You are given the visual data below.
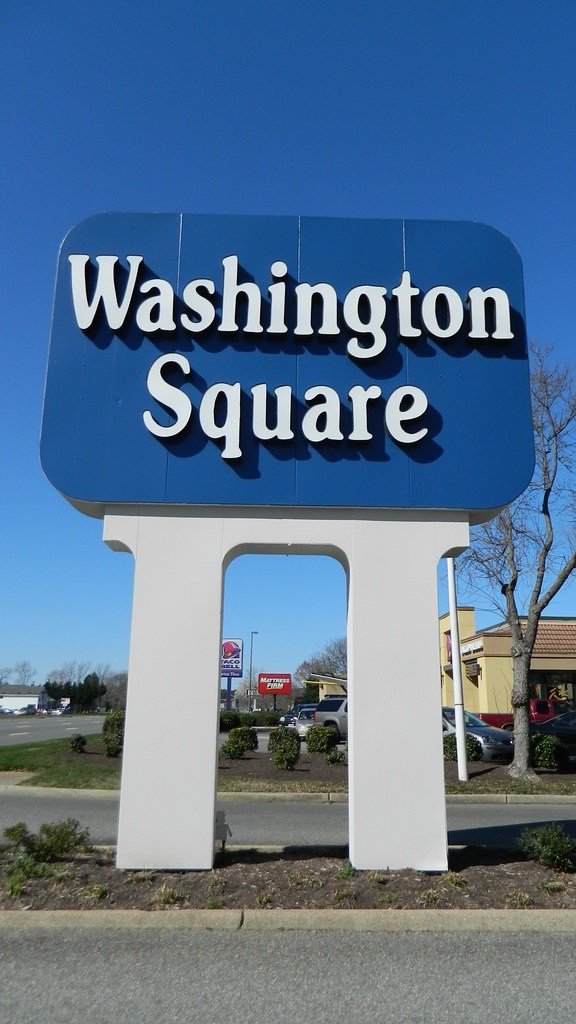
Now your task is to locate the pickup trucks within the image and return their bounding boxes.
[478,699,575,731]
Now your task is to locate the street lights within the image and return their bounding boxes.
[247,631,259,714]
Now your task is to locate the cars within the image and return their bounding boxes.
[295,708,317,740]
[442,706,514,762]
[16,707,36,715]
[246,706,252,711]
[530,709,576,758]
[3,709,13,713]
[313,694,348,744]
[37,706,74,716]
[279,713,296,726]
[296,703,318,716]
[267,707,279,712]
